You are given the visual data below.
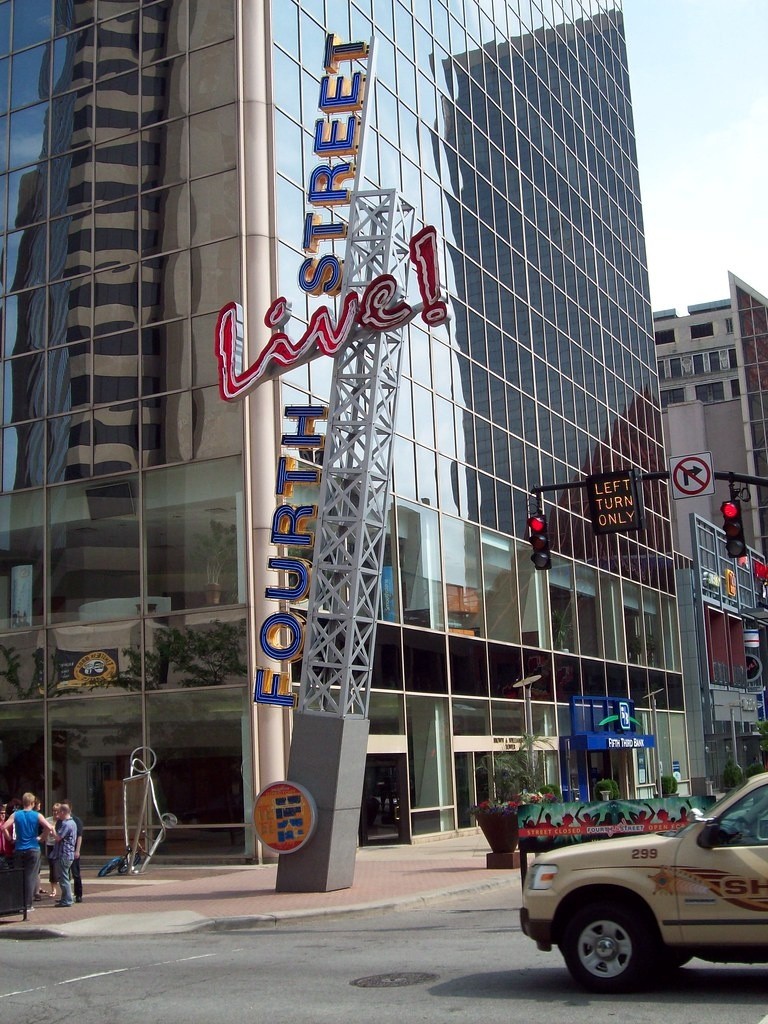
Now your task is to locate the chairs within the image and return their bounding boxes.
[77,598,141,621]
[129,596,172,616]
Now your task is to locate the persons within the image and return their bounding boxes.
[1,793,53,913]
[53,798,85,903]
[43,802,75,898]
[0,806,15,858]
[29,796,47,900]
[6,798,23,857]
[49,805,78,908]
[1,798,11,813]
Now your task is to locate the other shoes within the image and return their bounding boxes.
[55,899,72,908]
[76,897,82,902]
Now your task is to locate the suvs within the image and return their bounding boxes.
[513,768,768,991]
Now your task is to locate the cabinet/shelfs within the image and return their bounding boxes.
[104,778,152,851]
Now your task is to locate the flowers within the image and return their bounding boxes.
[464,767,560,816]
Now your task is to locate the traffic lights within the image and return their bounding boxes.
[528,513,554,573]
[721,497,751,562]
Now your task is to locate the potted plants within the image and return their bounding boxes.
[723,758,743,795]
[552,609,573,653]
[654,776,679,799]
[187,518,237,606]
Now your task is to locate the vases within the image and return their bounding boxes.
[473,813,520,854]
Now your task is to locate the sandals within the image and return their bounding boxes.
[49,893,56,897]
[33,894,42,900]
